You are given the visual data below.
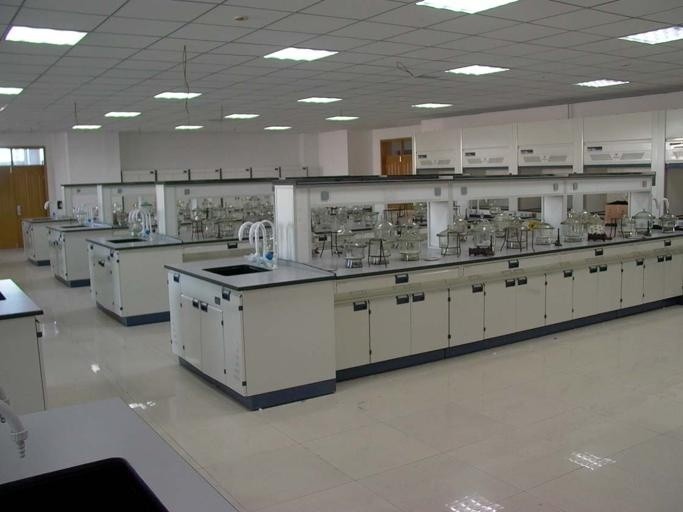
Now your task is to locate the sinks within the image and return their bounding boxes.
[107,238,148,243]
[202,264,272,276]
[32,218,49,221]
[62,225,89,227]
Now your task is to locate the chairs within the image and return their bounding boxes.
[336,235,533,269]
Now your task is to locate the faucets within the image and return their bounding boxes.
[248,222,267,259]
[128,209,150,238]
[238,221,260,252]
[255,219,278,269]
[44,201,57,218]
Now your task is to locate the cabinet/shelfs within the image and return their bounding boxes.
[165,269,335,410]
[0,317,47,414]
[542,258,574,336]
[477,262,548,352]
[21,221,71,267]
[445,273,485,358]
[47,229,113,288]
[567,248,621,330]
[336,293,370,382]
[617,243,683,316]
[87,242,183,326]
[368,283,451,374]
